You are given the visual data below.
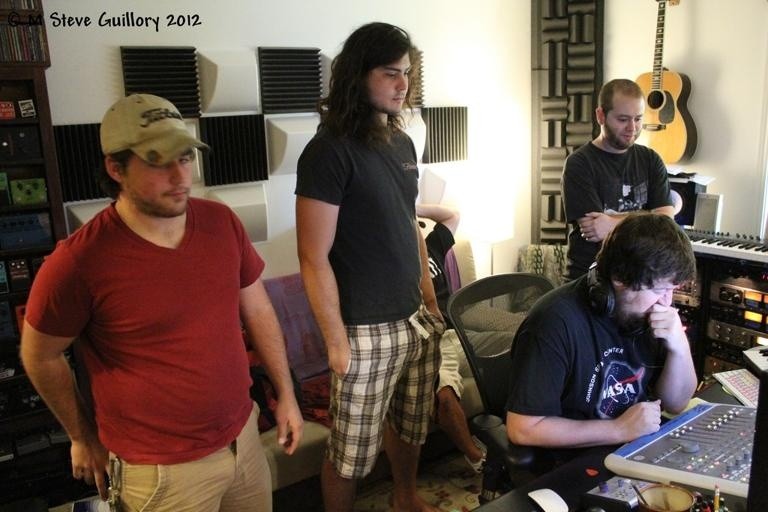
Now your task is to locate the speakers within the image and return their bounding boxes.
[692,193,725,234]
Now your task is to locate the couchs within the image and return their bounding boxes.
[237,237,529,492]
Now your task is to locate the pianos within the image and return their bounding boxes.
[682,224,768,269]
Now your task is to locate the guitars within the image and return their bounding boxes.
[635,0,698,165]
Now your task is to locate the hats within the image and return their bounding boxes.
[100,94,212,167]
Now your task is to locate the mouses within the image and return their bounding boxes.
[527,487,570,512]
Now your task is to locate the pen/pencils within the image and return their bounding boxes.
[689,485,726,512]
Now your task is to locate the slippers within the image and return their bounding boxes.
[464,452,487,474]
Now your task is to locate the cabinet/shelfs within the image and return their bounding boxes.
[0,69,109,511]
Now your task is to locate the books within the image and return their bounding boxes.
[0,0,47,66]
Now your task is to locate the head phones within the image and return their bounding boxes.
[585,261,616,318]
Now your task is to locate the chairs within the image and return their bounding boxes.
[445,271,557,503]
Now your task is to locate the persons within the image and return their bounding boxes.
[294,22,446,510]
[503,211,698,491]
[561,77,676,285]
[20,92,307,512]
[414,215,519,476]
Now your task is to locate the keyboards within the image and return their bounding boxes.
[712,368,760,407]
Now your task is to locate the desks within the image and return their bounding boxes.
[465,365,759,511]
